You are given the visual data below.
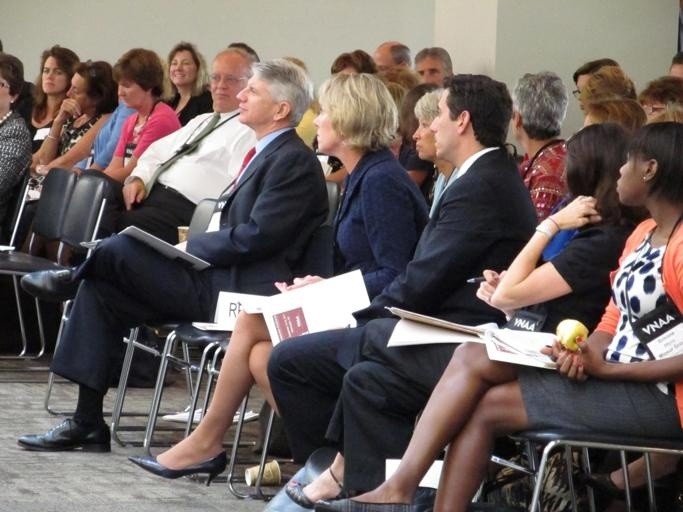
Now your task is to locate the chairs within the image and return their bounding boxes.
[112,181,338,446]
[46,198,228,432]
[256,408,276,501]
[201,338,274,498]
[0,175,33,358]
[0,169,112,382]
[444,430,683,512]
[0,167,78,371]
[147,323,295,463]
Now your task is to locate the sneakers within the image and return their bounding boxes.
[315,499,413,512]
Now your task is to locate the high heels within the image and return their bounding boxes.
[592,470,678,500]
[129,450,229,486]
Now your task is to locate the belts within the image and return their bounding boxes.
[160,182,184,196]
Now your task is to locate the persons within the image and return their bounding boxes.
[160,40,214,126]
[412,87,456,219]
[265,73,542,481]
[129,72,429,487]
[583,99,647,132]
[639,76,683,123]
[14,61,118,262]
[20,58,175,265]
[68,47,257,387]
[578,66,637,109]
[281,56,320,151]
[384,82,408,158]
[284,124,650,512]
[313,117,683,512]
[386,82,435,208]
[669,52,683,77]
[573,59,620,114]
[373,40,412,70]
[31,46,80,153]
[376,67,426,91]
[312,50,378,183]
[59,48,182,267]
[16,59,329,452]
[511,72,569,223]
[417,49,453,85]
[0,53,32,230]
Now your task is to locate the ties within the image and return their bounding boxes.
[235,149,255,189]
[141,112,219,199]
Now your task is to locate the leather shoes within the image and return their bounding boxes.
[18,417,112,453]
[22,264,85,304]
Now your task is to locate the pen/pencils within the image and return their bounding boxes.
[467,277,486,283]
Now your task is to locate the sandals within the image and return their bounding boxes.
[287,480,353,507]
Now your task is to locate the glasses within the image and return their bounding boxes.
[210,74,250,85]
[0,81,10,89]
[645,106,669,114]
[574,90,584,96]
[87,59,99,92]
